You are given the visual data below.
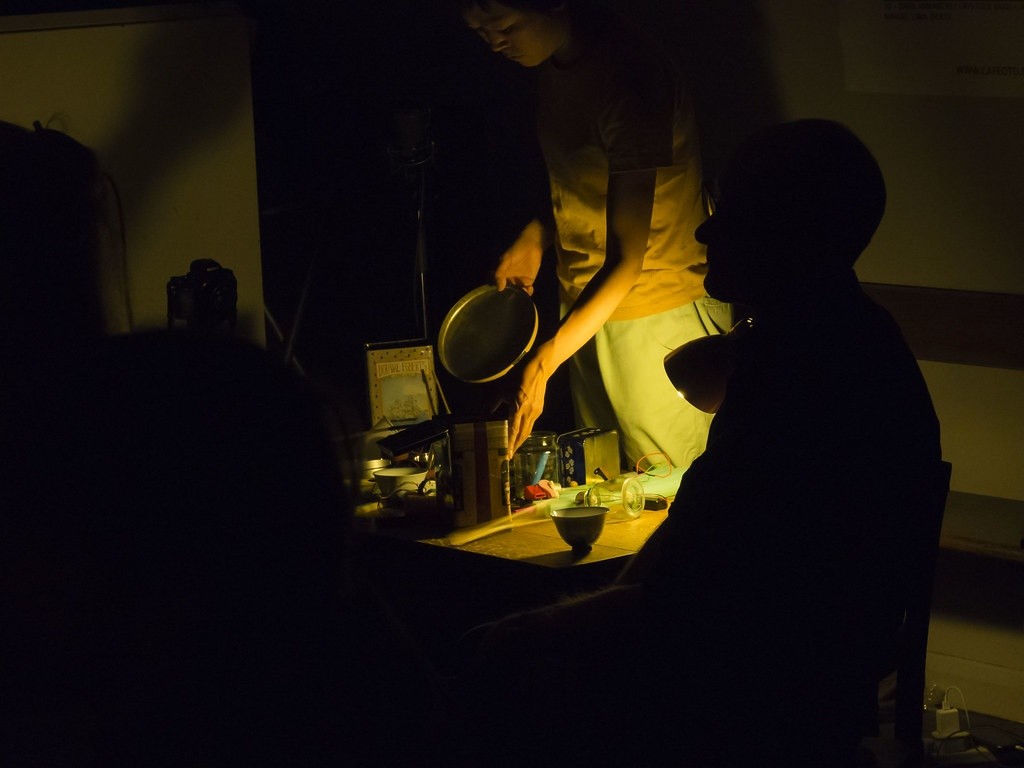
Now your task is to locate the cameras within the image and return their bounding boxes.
[166,258,238,329]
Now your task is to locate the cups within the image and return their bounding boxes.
[365,417,398,457]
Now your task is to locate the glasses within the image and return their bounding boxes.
[698,179,819,242]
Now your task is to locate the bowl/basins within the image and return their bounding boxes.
[341,459,390,500]
[549,507,610,555]
[373,467,429,499]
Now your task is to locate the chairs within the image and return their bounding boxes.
[713,461,951,768]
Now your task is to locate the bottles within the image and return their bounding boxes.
[584,478,645,519]
[514,432,564,499]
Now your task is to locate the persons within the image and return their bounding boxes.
[460,0,735,474]
[470,118,939,767]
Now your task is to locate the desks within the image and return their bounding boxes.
[348,469,677,581]
